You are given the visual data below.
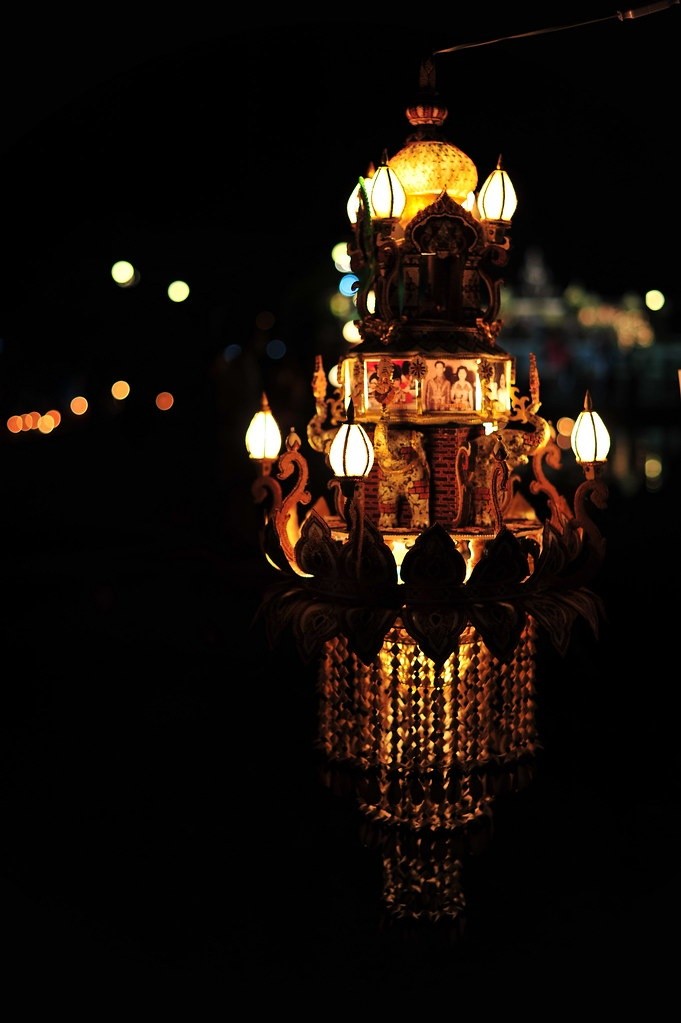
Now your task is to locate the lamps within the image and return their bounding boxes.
[245,62,612,918]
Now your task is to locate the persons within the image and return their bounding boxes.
[425,360,451,411]
[368,361,413,407]
[453,366,474,411]
[489,365,507,409]
